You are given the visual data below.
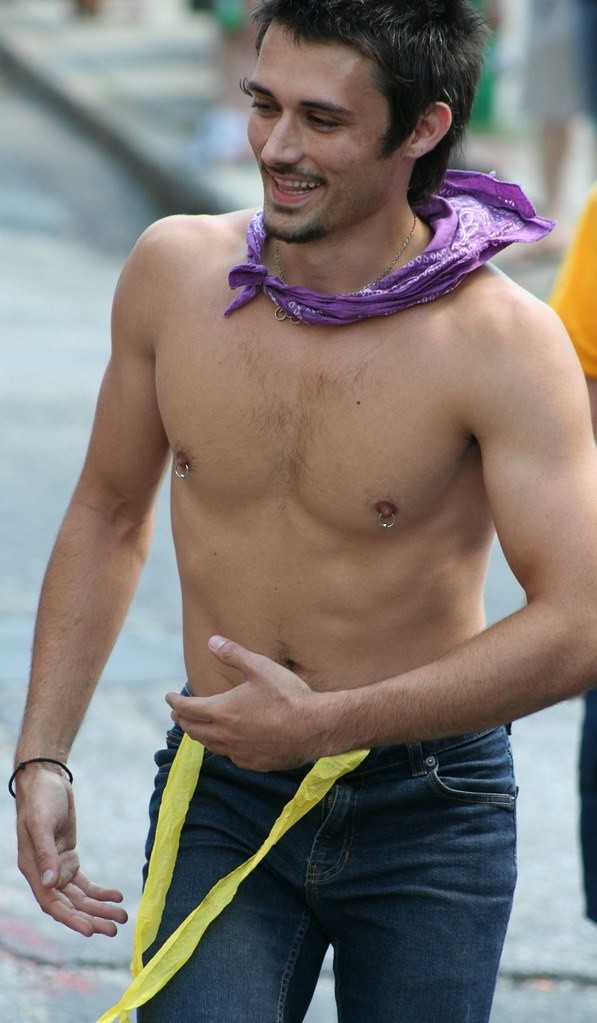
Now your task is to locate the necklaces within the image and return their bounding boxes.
[265,210,419,327]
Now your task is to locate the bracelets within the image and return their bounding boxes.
[6,757,75,796]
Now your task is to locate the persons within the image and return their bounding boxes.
[0,1,596,1023]
[520,1,577,208]
